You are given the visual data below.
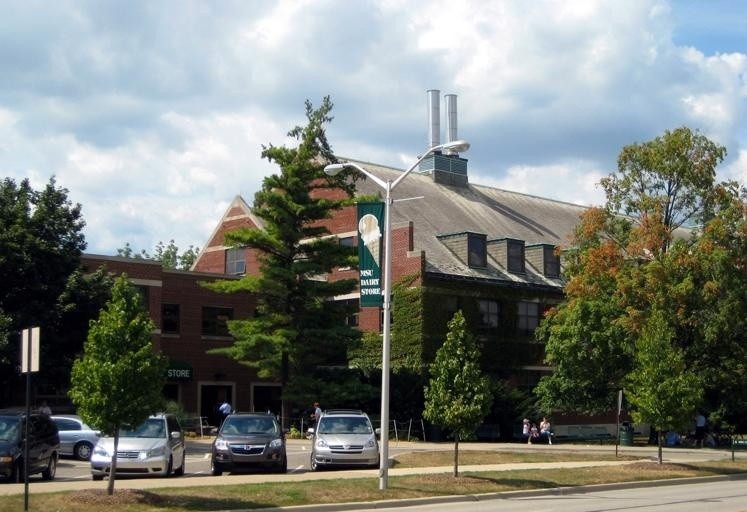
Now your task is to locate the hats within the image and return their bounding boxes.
[523,418,530,423]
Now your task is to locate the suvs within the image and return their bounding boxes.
[89,414,185,478]
[212,409,287,477]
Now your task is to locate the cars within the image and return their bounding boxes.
[307,409,381,471]
[49,415,106,459]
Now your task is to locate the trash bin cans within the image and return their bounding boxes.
[619,421,634,447]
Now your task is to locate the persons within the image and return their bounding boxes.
[530,423,540,438]
[693,410,706,448]
[522,419,532,445]
[703,433,715,448]
[310,402,322,423]
[38,400,52,416]
[539,417,554,445]
[218,398,231,425]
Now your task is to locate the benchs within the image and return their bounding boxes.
[512,424,620,446]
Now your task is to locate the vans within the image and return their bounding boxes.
[0,412,60,483]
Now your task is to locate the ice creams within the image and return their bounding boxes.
[358,214,381,267]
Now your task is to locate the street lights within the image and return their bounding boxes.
[322,139,471,491]
